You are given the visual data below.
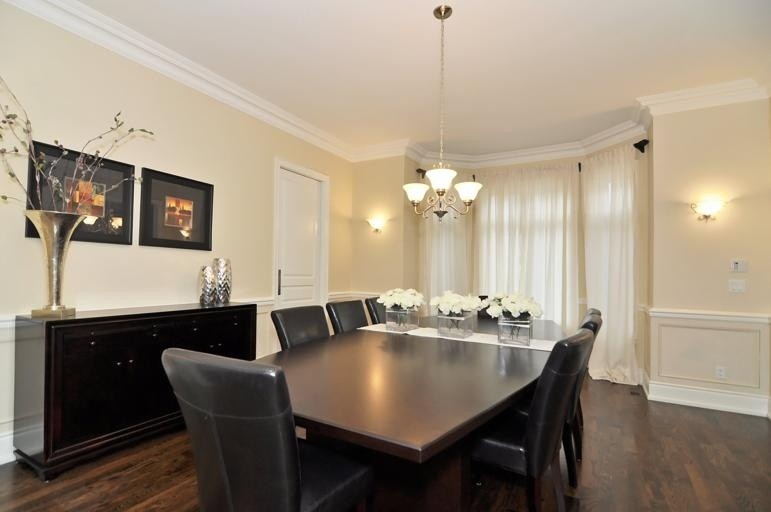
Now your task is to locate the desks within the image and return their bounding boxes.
[238,315,572,467]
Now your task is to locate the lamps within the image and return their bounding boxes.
[690,189,727,224]
[404,0,484,225]
[365,210,388,232]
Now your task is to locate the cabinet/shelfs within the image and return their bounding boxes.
[9,301,261,482]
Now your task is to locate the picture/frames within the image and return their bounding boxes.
[137,167,217,253]
[22,137,136,247]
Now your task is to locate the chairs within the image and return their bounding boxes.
[577,307,604,461]
[161,346,377,512]
[326,298,370,336]
[508,315,603,487]
[271,303,331,351]
[478,294,530,317]
[470,327,600,511]
[365,296,410,324]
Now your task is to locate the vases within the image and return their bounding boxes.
[498,317,535,346]
[437,315,475,339]
[25,208,90,320]
[385,309,422,333]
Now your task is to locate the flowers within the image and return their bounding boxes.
[377,286,427,312]
[482,292,545,319]
[429,289,483,316]
[0,75,158,214]
